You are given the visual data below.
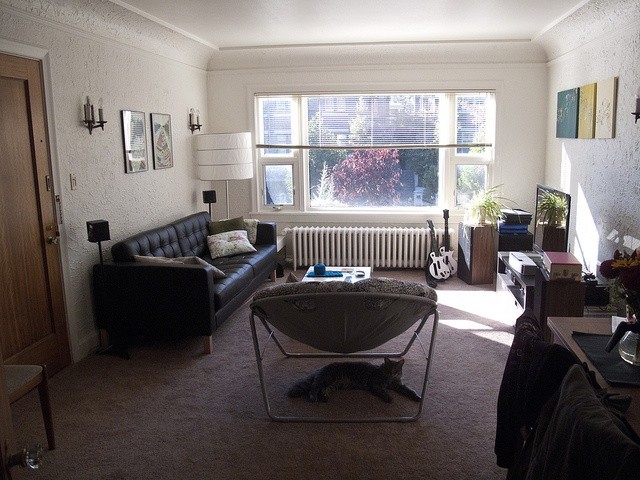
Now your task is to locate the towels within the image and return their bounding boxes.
[571,330,639,389]
[307,272,343,277]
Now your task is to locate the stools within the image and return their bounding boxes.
[2,364,56,451]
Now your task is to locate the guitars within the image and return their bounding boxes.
[426,219,451,281]
[438,209,458,275]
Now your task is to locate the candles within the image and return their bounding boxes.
[197,116,201,125]
[98,108,103,120]
[85,104,91,121]
[191,114,195,124]
[635,97,640,114]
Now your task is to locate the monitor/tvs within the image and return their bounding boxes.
[532,182,571,268]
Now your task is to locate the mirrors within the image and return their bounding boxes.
[532,184,572,252]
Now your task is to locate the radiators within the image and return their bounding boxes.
[282,226,455,272]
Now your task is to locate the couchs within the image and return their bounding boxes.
[93,212,277,355]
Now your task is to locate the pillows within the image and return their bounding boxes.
[206,216,244,235]
[133,255,184,265]
[218,219,260,245]
[207,229,257,260]
[161,256,228,280]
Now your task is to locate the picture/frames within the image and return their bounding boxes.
[121,110,149,174]
[150,113,173,170]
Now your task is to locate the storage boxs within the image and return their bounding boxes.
[542,251,582,281]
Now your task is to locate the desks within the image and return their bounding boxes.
[547,316,640,438]
[497,252,543,312]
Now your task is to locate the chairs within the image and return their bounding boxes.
[519,306,575,440]
[525,363,640,480]
[248,277,439,423]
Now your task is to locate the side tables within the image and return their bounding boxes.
[276,235,286,267]
[532,270,585,326]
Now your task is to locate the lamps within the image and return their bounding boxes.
[195,131,254,219]
[82,96,107,135]
[189,109,202,134]
[631,86,640,123]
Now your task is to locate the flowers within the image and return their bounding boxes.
[599,248,640,323]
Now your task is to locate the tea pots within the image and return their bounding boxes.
[604,321,640,366]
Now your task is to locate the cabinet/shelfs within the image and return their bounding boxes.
[457,221,497,285]
[534,224,565,250]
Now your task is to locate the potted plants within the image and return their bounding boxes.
[471,184,521,237]
[537,188,568,233]
